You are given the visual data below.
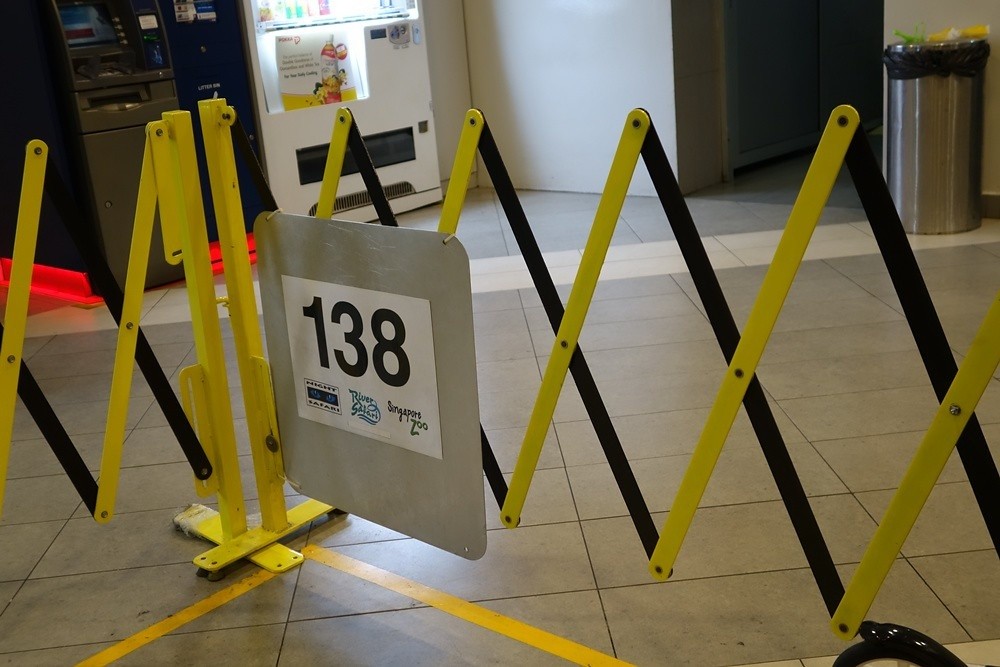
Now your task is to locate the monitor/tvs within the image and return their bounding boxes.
[57,4,121,47]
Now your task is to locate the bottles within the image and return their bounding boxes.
[256,0,406,22]
[318,33,342,104]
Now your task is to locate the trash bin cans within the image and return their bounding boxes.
[887,38,987,236]
